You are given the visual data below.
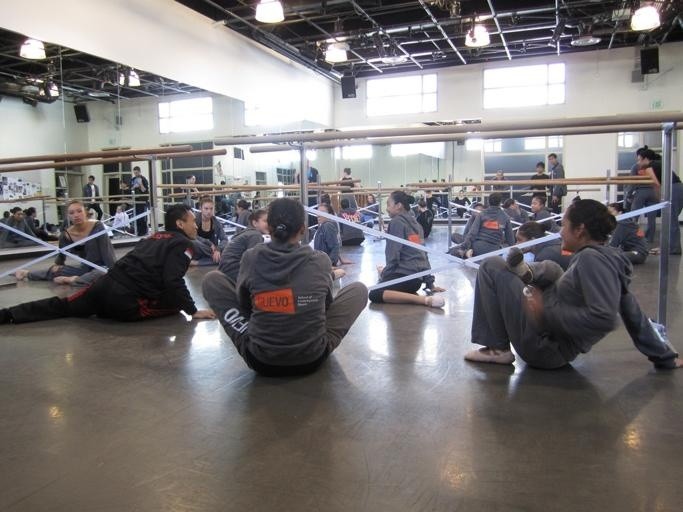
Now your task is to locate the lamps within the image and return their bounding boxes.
[39,82,60,97]
[325,44,348,63]
[255,0,284,23]
[120,71,141,87]
[571,23,601,46]
[465,24,490,47]
[630,6,660,31]
[19,39,46,59]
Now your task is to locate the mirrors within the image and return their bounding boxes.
[1,28,250,259]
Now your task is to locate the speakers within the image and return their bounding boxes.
[640,46,659,75]
[73,104,89,123]
[341,71,357,98]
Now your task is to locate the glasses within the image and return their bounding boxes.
[571,196,581,223]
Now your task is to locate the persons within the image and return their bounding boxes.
[0,204,216,322]
[465,201,683,370]
[201,197,368,378]
[1,143,683,273]
[218,210,270,281]
[369,191,446,308]
[16,198,117,287]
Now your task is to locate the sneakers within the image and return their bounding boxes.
[53,275,79,286]
[331,269,345,280]
[506,246,533,285]
[425,295,444,308]
[463,346,515,365]
